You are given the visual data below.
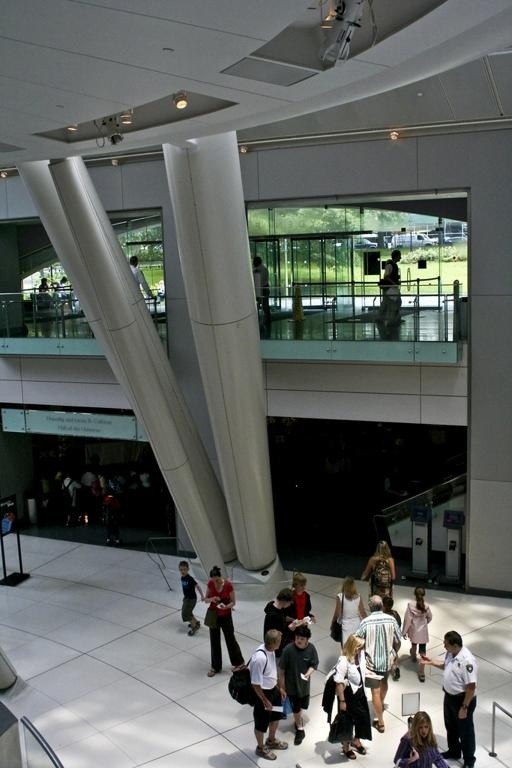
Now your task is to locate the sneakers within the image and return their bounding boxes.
[264,738,288,750]
[256,745,278,761]
[294,728,306,746]
[295,721,304,731]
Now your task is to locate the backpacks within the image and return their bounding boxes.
[372,556,392,588]
[61,479,73,506]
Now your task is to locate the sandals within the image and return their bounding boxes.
[374,722,384,733]
[188,622,201,628]
[189,625,199,636]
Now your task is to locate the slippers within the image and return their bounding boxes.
[351,741,367,755]
[340,748,356,759]
[207,668,219,677]
[232,663,246,672]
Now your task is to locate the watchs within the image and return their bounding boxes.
[462,704,469,709]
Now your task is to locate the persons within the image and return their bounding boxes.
[393,710,452,768]
[361,541,396,601]
[401,586,432,683]
[321,633,372,760]
[139,469,151,489]
[263,588,300,720]
[252,257,271,333]
[417,631,479,768]
[203,565,246,678]
[248,630,288,761]
[284,572,318,643]
[352,595,401,733]
[278,625,319,745]
[381,596,401,681]
[383,250,406,323]
[330,576,366,664]
[80,464,96,487]
[128,255,156,301]
[101,487,123,546]
[39,278,48,293]
[178,560,205,636]
[51,276,68,305]
[62,477,81,528]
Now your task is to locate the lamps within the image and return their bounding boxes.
[174,90,187,110]
[388,132,399,142]
[122,112,133,126]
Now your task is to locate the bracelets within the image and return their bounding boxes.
[339,700,346,703]
